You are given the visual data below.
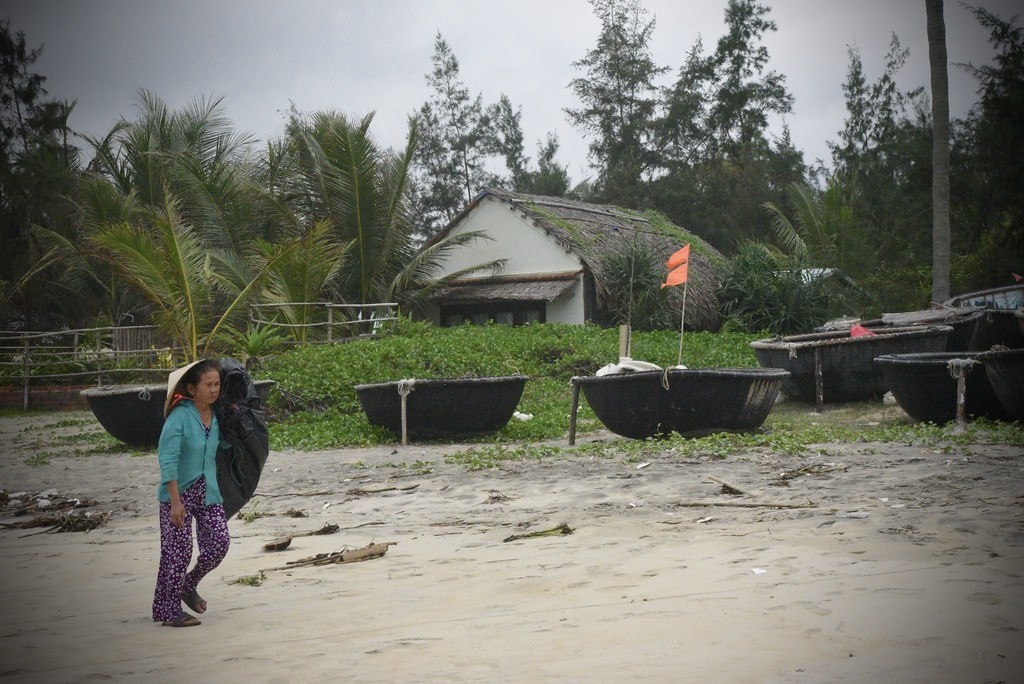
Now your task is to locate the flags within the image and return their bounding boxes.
[660,263,687,288]
[667,244,689,268]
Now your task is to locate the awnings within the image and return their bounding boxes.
[394,278,578,302]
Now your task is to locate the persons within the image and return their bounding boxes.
[152,358,231,627]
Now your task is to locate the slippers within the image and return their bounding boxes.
[181,590,206,614]
[161,612,201,627]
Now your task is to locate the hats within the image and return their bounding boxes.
[164,359,207,419]
